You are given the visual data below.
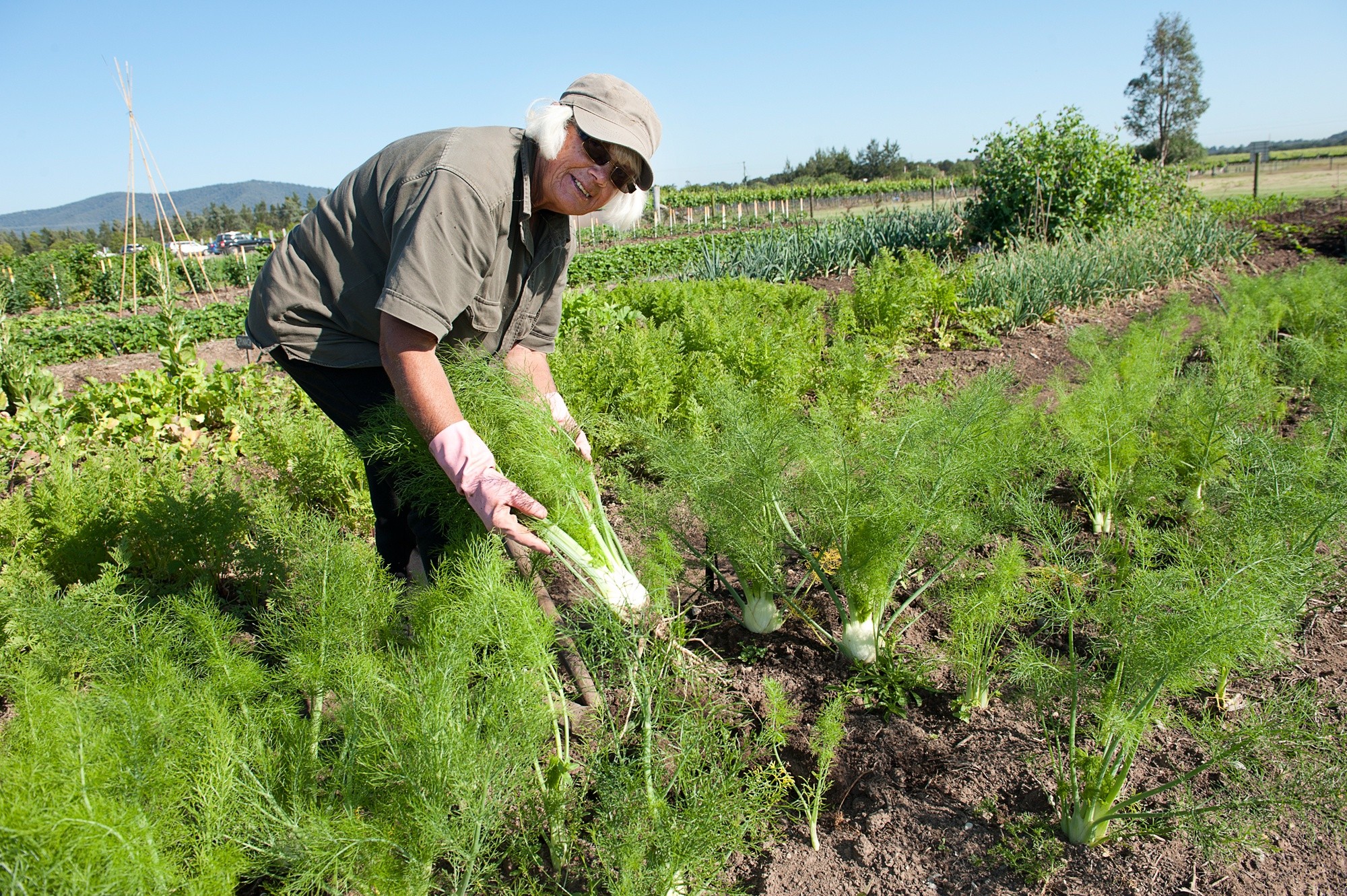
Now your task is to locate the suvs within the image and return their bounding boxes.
[166,240,209,256]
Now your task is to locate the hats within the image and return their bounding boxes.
[560,74,661,191]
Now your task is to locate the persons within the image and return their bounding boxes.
[244,72,665,653]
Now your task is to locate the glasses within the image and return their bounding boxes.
[570,120,637,194]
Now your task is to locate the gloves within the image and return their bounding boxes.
[429,419,551,555]
[533,392,592,464]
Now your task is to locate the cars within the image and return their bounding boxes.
[93,246,117,257]
[120,243,145,254]
[206,231,275,255]
